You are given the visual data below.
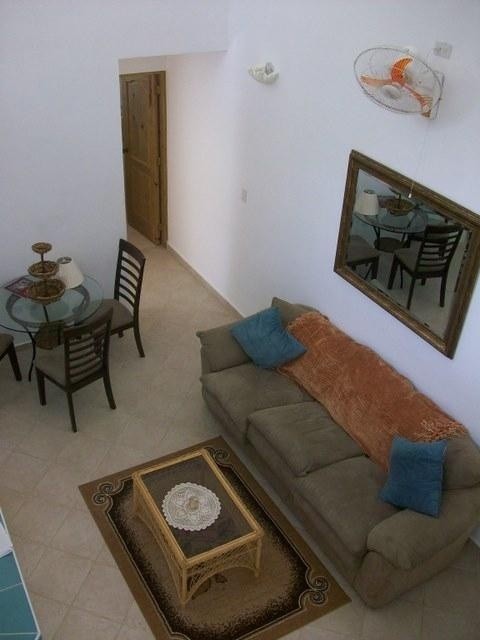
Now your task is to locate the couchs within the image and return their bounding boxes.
[196,304,480,610]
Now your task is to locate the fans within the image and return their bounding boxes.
[354,46,446,119]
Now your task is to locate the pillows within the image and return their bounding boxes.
[378,435,448,519]
[229,306,307,370]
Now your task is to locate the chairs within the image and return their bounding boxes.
[0,239,145,433]
[346,209,464,310]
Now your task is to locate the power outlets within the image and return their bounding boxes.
[434,42,451,59]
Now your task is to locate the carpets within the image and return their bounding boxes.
[78,436,352,640]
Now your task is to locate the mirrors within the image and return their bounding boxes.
[333,150,480,360]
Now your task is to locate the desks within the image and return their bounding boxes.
[0,273,104,382]
[353,196,429,289]
